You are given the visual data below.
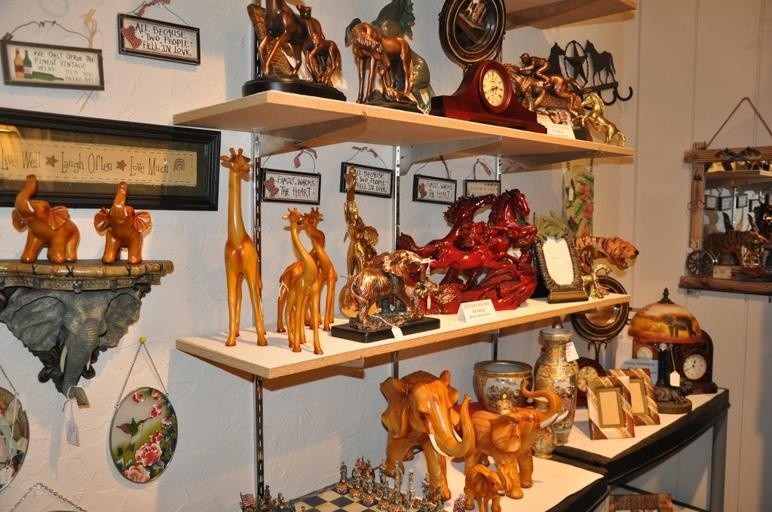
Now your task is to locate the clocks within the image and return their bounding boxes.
[655,335,718,396]
[570,273,630,369]
[575,356,607,408]
[429,60,547,134]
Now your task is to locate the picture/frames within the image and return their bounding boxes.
[413,174,457,205]
[0,107,222,211]
[608,367,660,427]
[463,179,501,198]
[263,168,321,205]
[0,387,30,492]
[118,12,201,65]
[585,375,635,440]
[340,162,393,198]
[0,40,104,90]
[678,141,772,295]
[109,386,178,484]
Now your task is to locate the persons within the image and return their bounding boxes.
[519,52,552,85]
[338,457,445,512]
[260,485,305,512]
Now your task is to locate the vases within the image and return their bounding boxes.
[533,328,578,444]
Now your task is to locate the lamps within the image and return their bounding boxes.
[627,287,702,414]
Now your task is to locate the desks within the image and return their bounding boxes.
[283,373,731,512]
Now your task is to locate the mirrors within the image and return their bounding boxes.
[438,0,507,69]
[534,227,589,303]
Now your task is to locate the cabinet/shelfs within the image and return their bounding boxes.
[173,86,634,390]
